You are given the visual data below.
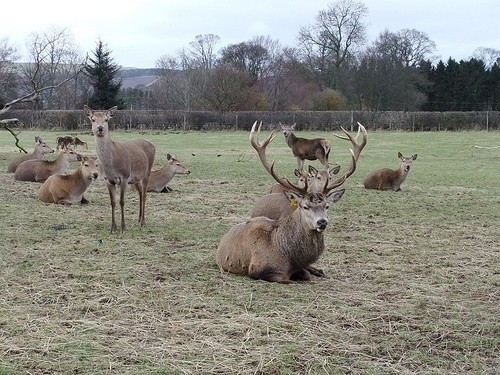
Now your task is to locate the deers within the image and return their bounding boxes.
[56,136,89,153]
[15,145,85,182]
[83,104,156,234]
[364,152,418,191]
[37,154,100,205]
[246,120,370,221]
[7,135,54,174]
[214,190,347,284]
[131,151,189,194]
